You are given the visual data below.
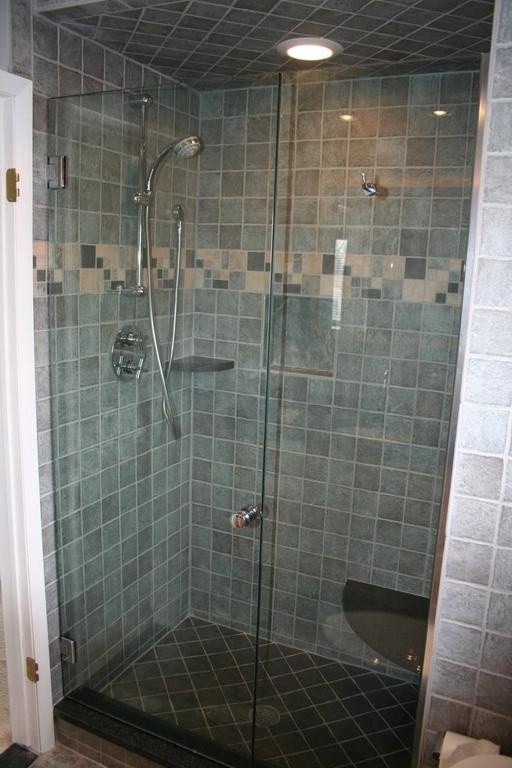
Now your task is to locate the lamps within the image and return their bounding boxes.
[277,37,344,61]
